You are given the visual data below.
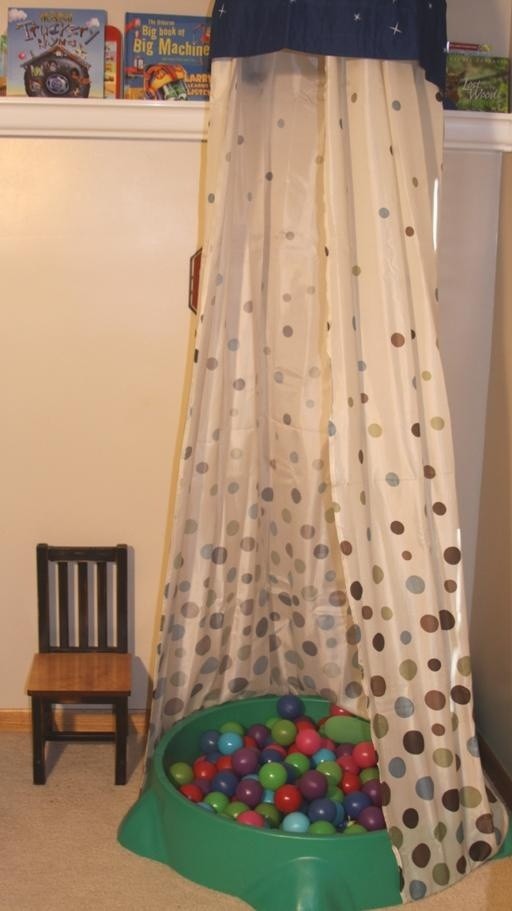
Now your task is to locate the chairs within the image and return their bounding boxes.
[22,541,133,785]
